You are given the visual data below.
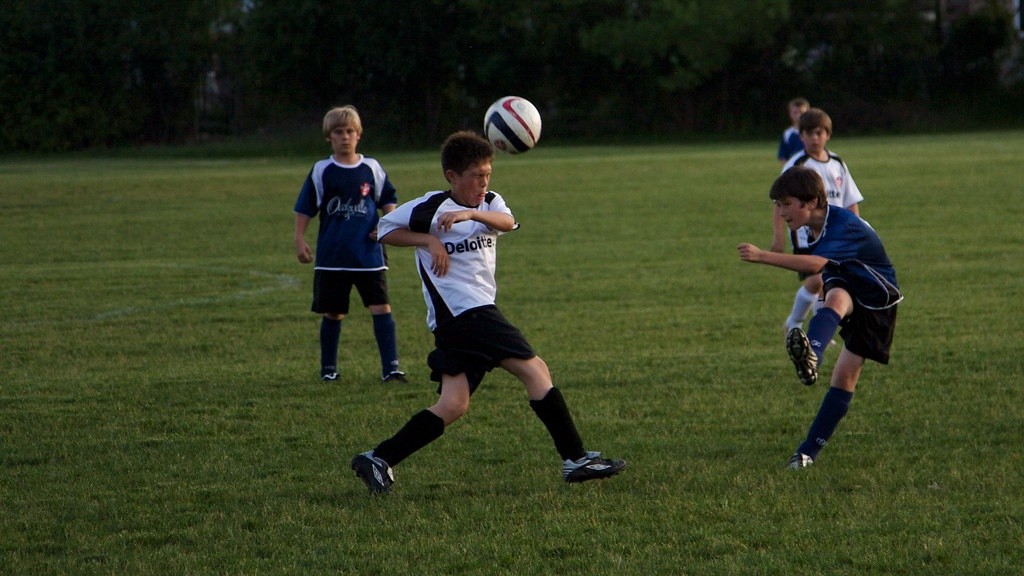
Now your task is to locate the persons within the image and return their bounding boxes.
[738,166,904,470]
[353,132,626,496]
[771,108,865,345]
[778,97,811,164]
[293,106,406,381]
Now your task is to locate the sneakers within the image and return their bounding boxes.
[320,365,340,380]
[382,360,407,384]
[562,451,626,484]
[787,327,819,386]
[351,449,395,494]
[790,450,820,467]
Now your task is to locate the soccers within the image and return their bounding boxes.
[484,96,542,155]
